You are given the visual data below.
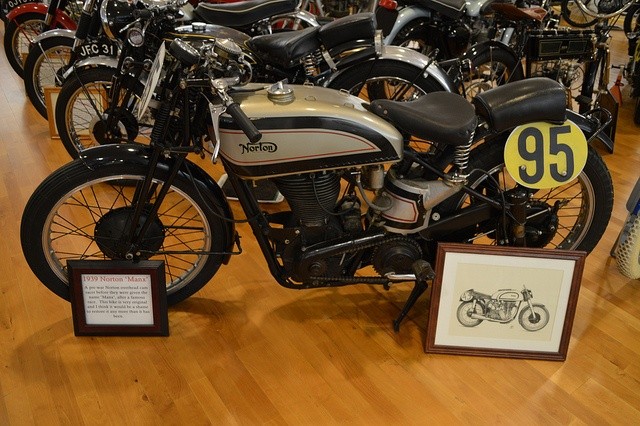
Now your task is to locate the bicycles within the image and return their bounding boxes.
[560,0,600,26]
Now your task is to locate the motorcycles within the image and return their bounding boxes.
[447,0,632,114]
[5,0,245,80]
[624,0,640,126]
[366,0,497,102]
[610,177,640,280]
[54,0,458,185]
[457,285,549,332]
[24,0,334,121]
[21,42,613,334]
